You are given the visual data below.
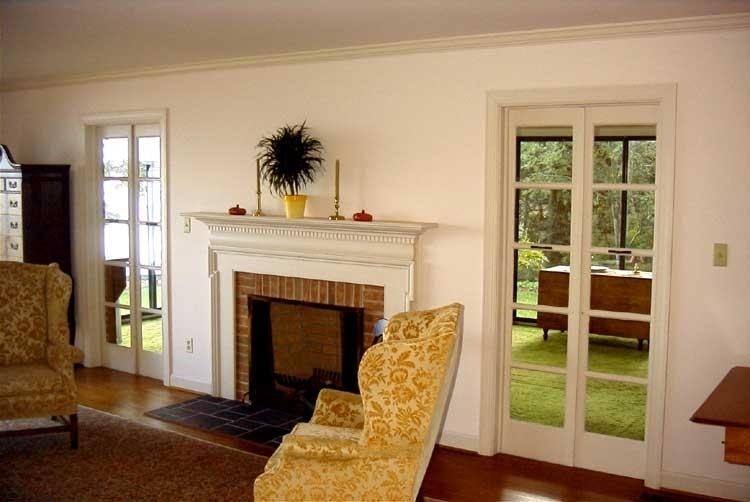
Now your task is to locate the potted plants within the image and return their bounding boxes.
[254,119,327,219]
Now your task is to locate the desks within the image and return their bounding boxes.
[689,366,750,466]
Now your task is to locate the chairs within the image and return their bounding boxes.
[0,260,84,451]
[252,303,464,502]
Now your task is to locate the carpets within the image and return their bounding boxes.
[144,394,310,449]
[0,404,450,502]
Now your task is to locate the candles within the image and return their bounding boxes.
[335,160,339,198]
[256,158,260,192]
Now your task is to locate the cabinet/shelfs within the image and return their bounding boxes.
[0,144,76,346]
[537,265,652,341]
